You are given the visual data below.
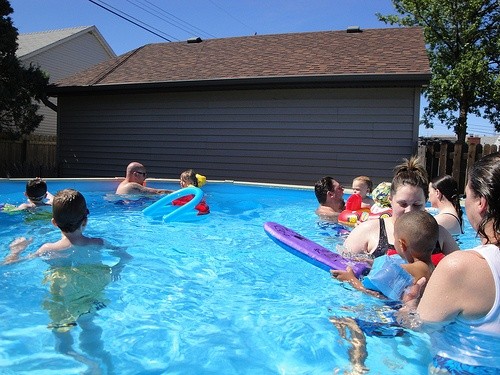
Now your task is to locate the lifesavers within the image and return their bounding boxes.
[141,187,204,218]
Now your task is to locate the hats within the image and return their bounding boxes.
[372,182,392,205]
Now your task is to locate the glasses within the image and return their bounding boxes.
[132,170,145,177]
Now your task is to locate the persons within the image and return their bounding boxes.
[344,152,459,257]
[429,173,465,234]
[180,169,207,188]
[4,188,133,375]
[330,210,439,300]
[402,153,500,375]
[351,176,374,208]
[16,176,56,210]
[314,176,346,218]
[115,162,177,197]
